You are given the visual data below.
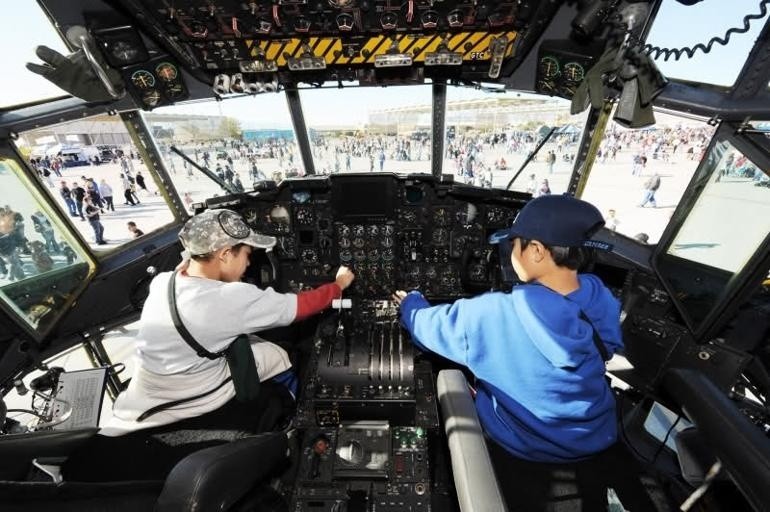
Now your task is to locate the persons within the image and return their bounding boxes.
[391,195,625,465]
[604,205,619,231]
[635,230,650,244]
[552,116,770,188]
[122,208,353,435]
[1,204,80,279]
[637,171,662,209]
[31,95,555,247]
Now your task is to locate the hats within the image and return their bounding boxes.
[488,195,614,251]
[178,208,276,254]
